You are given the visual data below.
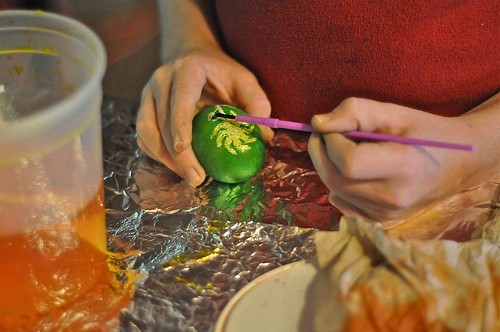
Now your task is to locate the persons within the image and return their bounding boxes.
[134,0,500,220]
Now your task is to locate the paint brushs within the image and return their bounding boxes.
[213,113,476,153]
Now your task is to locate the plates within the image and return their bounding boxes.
[212,255,321,332]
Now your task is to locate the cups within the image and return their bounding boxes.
[0,10,116,331]
